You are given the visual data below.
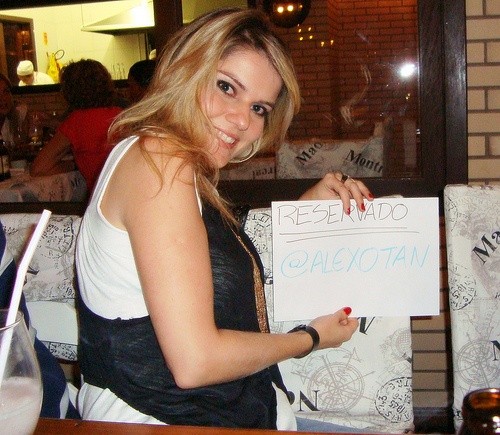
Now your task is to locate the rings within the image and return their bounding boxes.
[340,173,350,184]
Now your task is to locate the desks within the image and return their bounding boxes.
[0,139,88,203]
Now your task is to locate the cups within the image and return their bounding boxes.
[0,308,44,435]
[13,111,59,152]
[461,387,500,435]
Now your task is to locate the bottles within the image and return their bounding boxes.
[0,133,11,182]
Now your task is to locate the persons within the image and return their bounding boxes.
[0,219,83,419]
[75,7,373,433]
[0,48,157,216]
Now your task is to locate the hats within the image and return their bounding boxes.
[17,60,34,76]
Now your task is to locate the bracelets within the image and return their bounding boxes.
[286,323,319,359]
